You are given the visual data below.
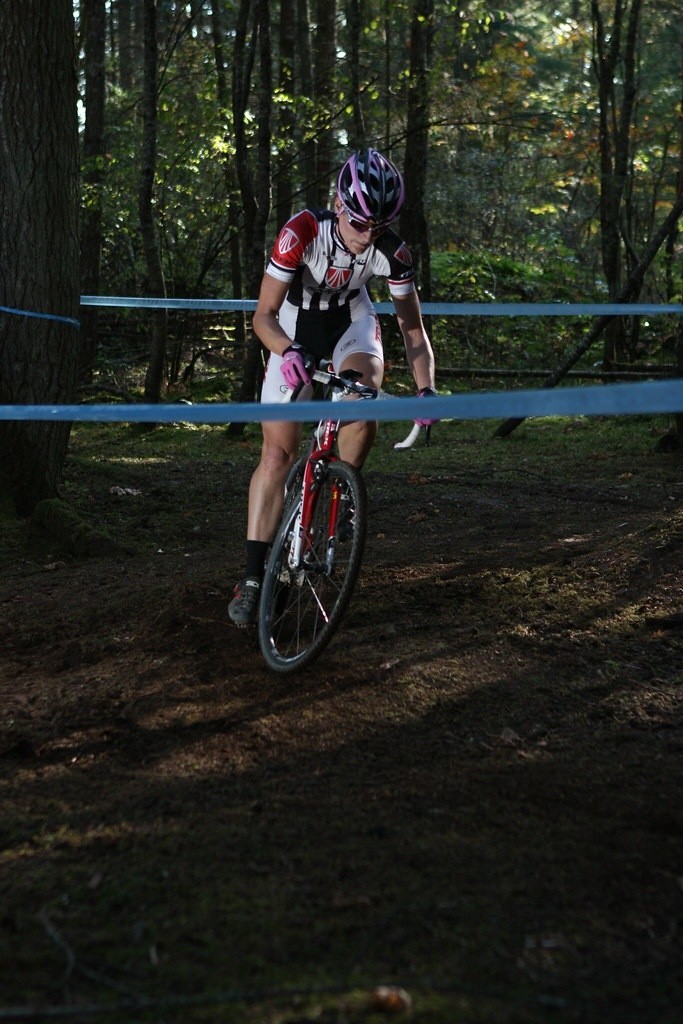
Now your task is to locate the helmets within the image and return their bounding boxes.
[337,147,405,225]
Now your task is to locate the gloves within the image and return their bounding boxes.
[280,343,310,390]
[414,386,440,427]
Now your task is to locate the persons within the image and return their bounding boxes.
[228,147,436,625]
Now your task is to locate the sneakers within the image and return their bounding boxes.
[337,504,355,543]
[228,577,261,622]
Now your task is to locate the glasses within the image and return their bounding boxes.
[345,209,389,234]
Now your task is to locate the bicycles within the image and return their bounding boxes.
[252,354,441,673]
